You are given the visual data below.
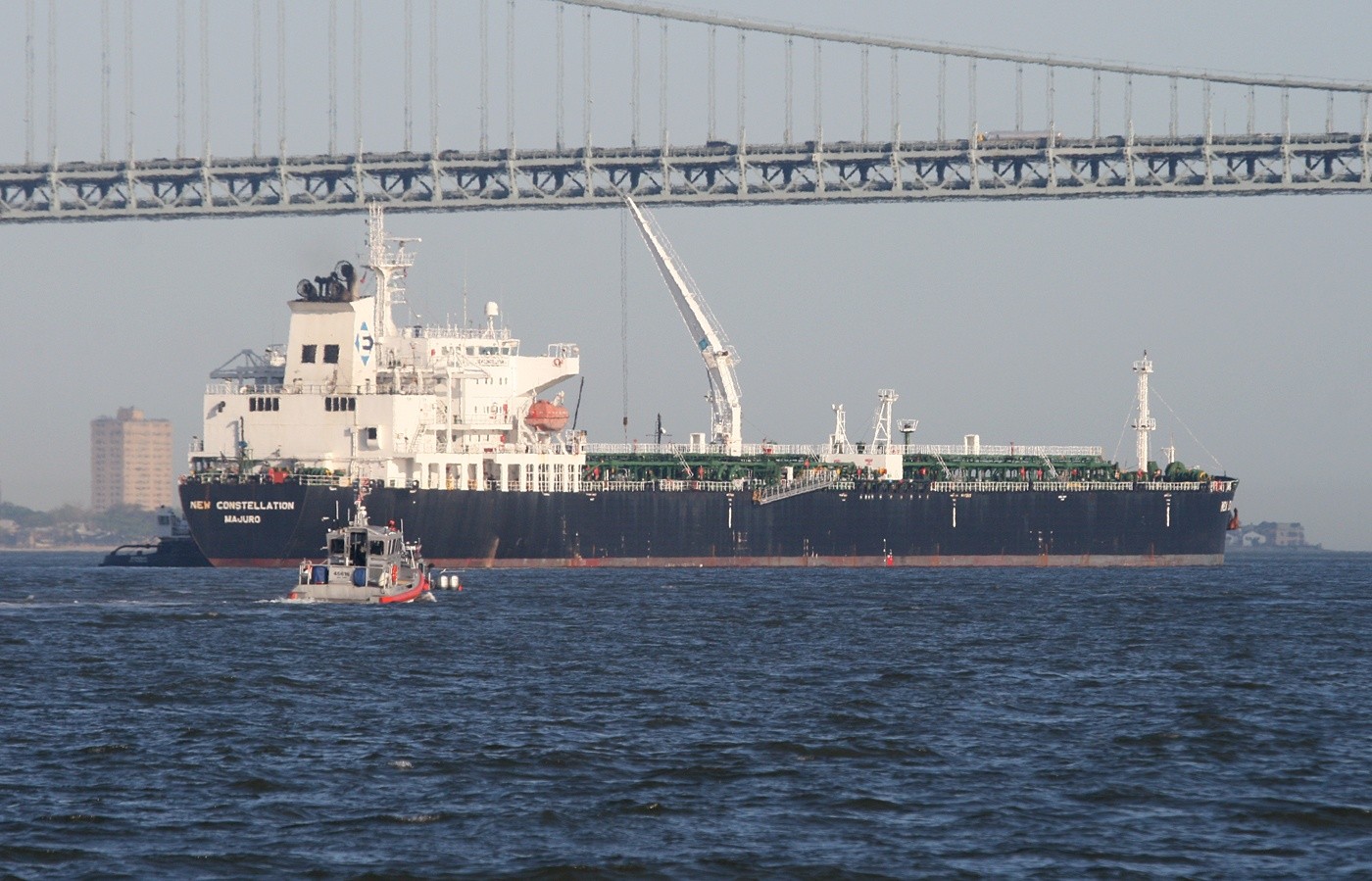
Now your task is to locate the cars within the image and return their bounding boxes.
[61,132,1372,169]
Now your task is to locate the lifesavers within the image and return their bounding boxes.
[554,358,561,366]
[391,563,398,584]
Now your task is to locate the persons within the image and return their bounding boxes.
[414,323,422,338]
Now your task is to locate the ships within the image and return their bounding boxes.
[177,202,1244,569]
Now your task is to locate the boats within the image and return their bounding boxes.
[522,400,569,431]
[288,477,431,605]
